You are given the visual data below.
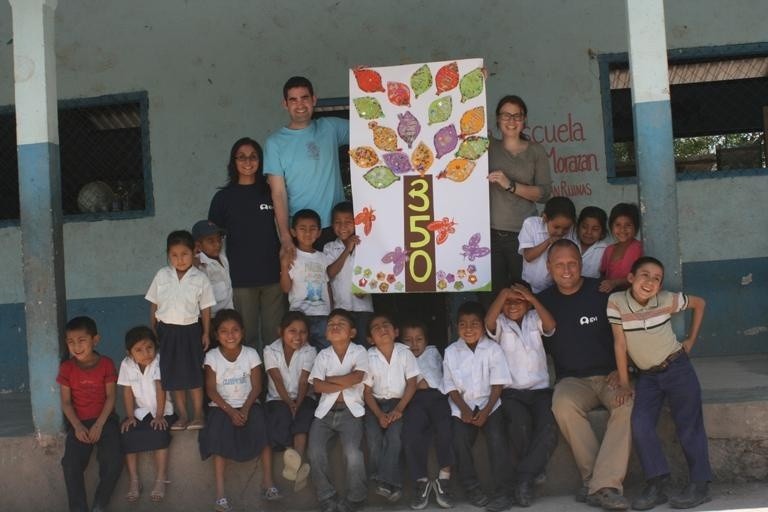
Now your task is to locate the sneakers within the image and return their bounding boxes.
[574,485,588,502]
[585,487,629,511]
[335,498,368,512]
[408,477,433,510]
[432,477,457,508]
[373,481,403,503]
[460,474,537,512]
[319,492,340,512]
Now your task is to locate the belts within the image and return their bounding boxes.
[643,347,684,374]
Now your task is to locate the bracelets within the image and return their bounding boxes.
[505,181,514,191]
[509,183,516,193]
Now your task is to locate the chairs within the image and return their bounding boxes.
[714,141,763,171]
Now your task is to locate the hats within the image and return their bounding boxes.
[191,220,227,242]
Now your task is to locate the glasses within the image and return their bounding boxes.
[232,153,260,162]
[497,112,526,121]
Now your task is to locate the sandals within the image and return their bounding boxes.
[292,462,311,493]
[124,479,143,502]
[171,419,204,431]
[150,478,172,501]
[260,487,284,501]
[281,447,302,480]
[212,497,233,512]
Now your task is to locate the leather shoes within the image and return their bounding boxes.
[631,481,669,510]
[667,481,712,510]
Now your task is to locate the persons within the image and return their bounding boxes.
[206,136,289,368]
[262,75,350,258]
[481,66,551,315]
